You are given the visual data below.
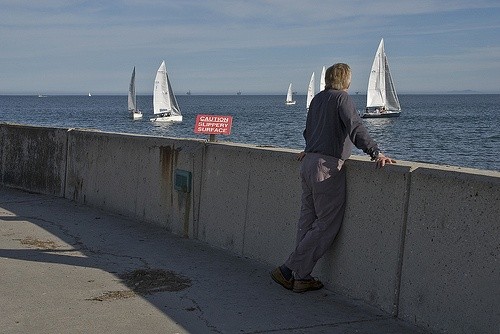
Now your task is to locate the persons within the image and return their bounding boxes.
[271,63,397,293]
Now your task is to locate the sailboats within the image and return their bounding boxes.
[285,84,297,105]
[320,66,328,92]
[305,72,315,111]
[359,39,403,118]
[127,65,142,120]
[150,59,183,122]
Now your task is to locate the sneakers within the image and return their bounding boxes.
[293,277,324,293]
[270,266,295,290]
